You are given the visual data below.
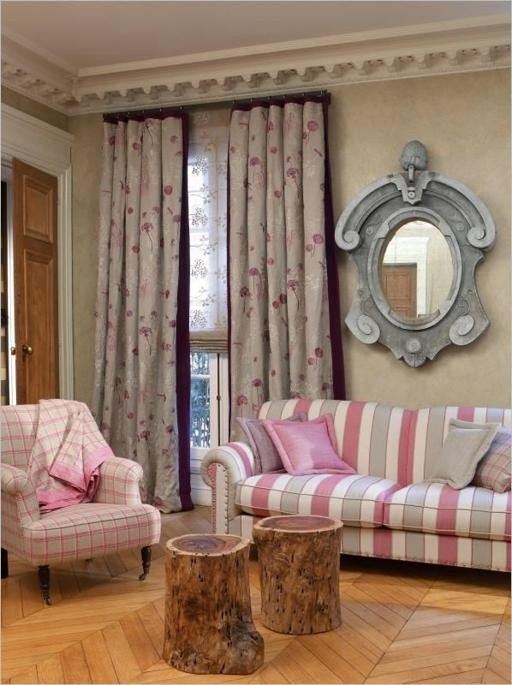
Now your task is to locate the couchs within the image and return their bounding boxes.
[205,393,509,572]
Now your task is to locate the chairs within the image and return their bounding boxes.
[1,398,161,604]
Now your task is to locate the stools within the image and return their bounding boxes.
[161,535,266,676]
[255,514,344,634]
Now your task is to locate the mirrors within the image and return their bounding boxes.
[337,142,495,372]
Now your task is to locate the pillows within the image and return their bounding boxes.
[237,414,277,475]
[428,415,497,488]
[271,412,355,476]
[472,425,508,490]
